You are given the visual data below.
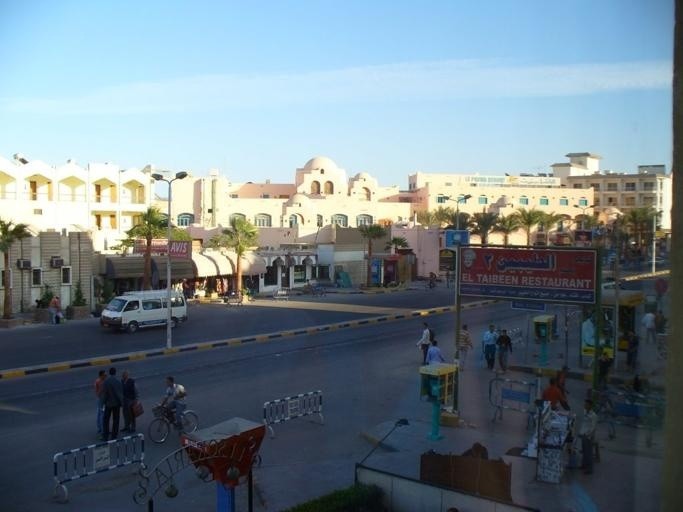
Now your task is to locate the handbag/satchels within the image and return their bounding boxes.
[130,399,144,418]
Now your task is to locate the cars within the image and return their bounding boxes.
[602,277,625,288]
[648,257,664,265]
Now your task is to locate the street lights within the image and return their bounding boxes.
[149,171,189,348]
[574,203,596,230]
[454,194,473,310]
[649,210,663,273]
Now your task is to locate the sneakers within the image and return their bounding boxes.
[576,464,593,475]
[167,418,185,431]
[95,426,137,441]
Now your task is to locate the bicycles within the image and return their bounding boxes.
[147,402,200,444]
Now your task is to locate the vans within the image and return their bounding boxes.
[100,289,187,332]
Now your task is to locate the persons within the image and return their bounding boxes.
[128,302,139,310]
[93,366,188,441]
[48,295,61,324]
[427,272,437,290]
